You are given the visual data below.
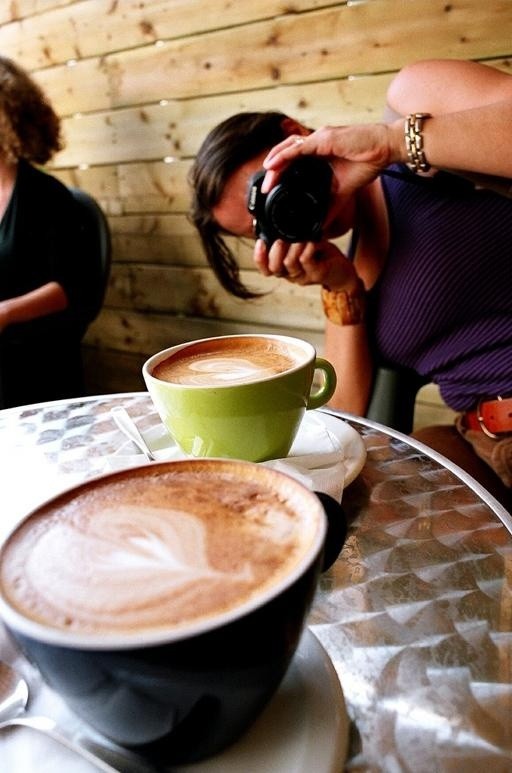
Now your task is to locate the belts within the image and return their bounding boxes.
[455,396,512,440]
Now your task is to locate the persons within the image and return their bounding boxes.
[2,53,115,411]
[182,60,512,520]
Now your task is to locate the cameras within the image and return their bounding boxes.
[247,156,334,248]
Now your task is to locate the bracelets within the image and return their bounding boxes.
[404,112,431,172]
[317,276,367,326]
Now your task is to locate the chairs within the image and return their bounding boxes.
[67,187,113,347]
[364,367,435,436]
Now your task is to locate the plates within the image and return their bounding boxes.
[1,629,352,773]
[103,410,368,493]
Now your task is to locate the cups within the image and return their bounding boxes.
[140,333,339,465]
[0,456,329,768]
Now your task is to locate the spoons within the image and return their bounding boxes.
[0,661,162,773]
